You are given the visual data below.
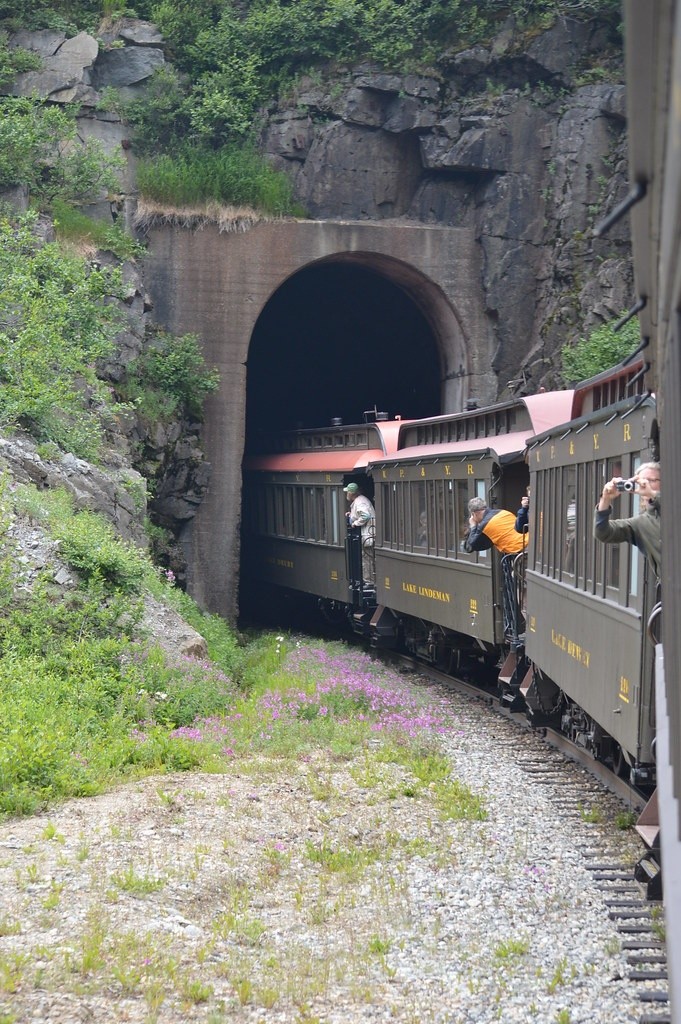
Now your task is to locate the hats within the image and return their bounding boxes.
[343,483,358,493]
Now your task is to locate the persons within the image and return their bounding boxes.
[592,462,661,588]
[343,483,375,590]
[417,512,427,547]
[465,497,528,641]
[514,486,530,534]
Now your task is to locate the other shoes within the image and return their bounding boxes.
[507,633,526,641]
[349,583,374,590]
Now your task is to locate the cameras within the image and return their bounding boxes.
[617,481,635,492]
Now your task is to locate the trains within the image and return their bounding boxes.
[244,0,681,1024]
